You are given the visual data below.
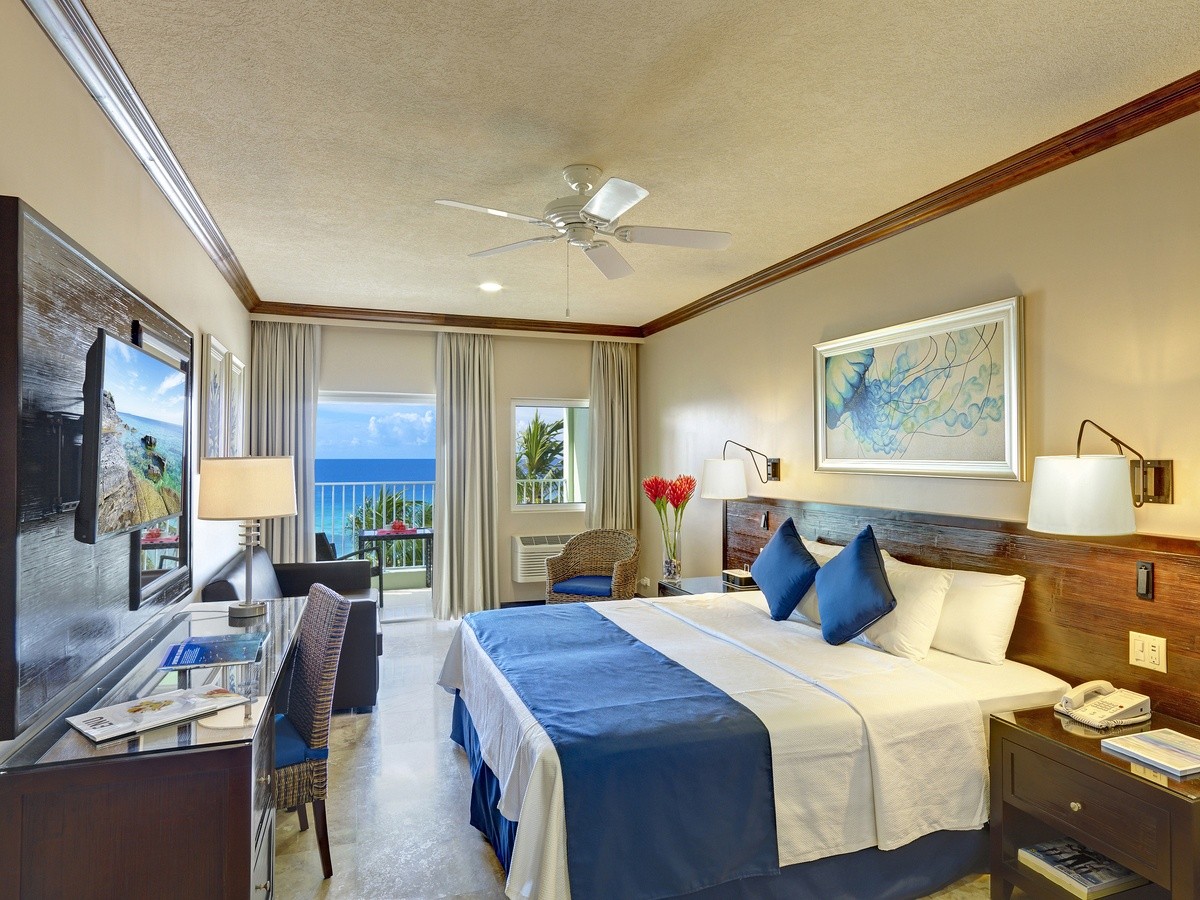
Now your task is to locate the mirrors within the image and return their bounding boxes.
[128,319,191,610]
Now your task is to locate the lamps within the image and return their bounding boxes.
[1026,418,1174,538]
[198,453,298,619]
[701,440,782,500]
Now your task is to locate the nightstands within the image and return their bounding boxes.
[657,575,762,597]
[989,701,1200,900]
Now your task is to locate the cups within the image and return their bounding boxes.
[159,532,165,539]
[385,525,392,530]
[404,524,409,530]
[143,532,148,537]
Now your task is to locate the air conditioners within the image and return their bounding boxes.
[510,533,579,584]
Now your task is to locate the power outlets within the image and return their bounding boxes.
[1128,630,1168,674]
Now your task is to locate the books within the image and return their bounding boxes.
[1101,727,1200,777]
[64,681,252,742]
[156,629,271,669]
[1016,837,1141,900]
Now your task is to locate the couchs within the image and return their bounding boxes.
[200,547,383,714]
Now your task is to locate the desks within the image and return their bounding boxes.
[358,527,433,589]
[0,596,308,900]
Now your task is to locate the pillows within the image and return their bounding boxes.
[757,545,833,625]
[854,566,955,660]
[800,534,847,558]
[880,549,1027,664]
[814,524,898,646]
[751,516,822,621]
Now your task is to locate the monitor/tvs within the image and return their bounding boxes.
[73,328,189,545]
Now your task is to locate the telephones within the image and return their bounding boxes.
[1052,679,1152,730]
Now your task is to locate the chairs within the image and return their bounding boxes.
[269,582,353,880]
[315,531,385,609]
[543,528,641,605]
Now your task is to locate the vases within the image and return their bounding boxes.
[661,530,683,580]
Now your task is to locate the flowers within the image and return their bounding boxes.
[641,472,697,561]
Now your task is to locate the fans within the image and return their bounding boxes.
[432,164,738,316]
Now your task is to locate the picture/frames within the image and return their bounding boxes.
[229,352,246,458]
[199,331,228,474]
[811,294,1028,484]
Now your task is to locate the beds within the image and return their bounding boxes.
[431,498,1200,900]
